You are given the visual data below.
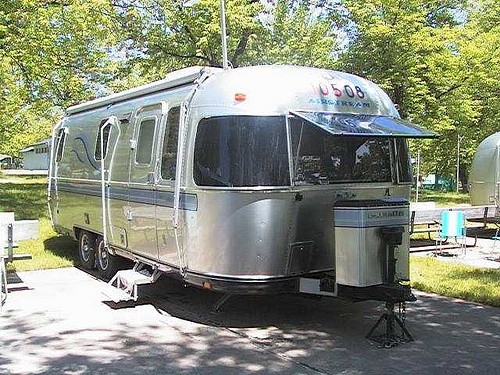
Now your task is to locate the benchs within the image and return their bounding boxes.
[410,204,500,247]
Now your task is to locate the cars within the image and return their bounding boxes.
[0,154,24,169]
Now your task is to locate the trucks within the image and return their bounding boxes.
[18,139,51,170]
[47,64,441,311]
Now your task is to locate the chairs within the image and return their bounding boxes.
[436,211,466,257]
